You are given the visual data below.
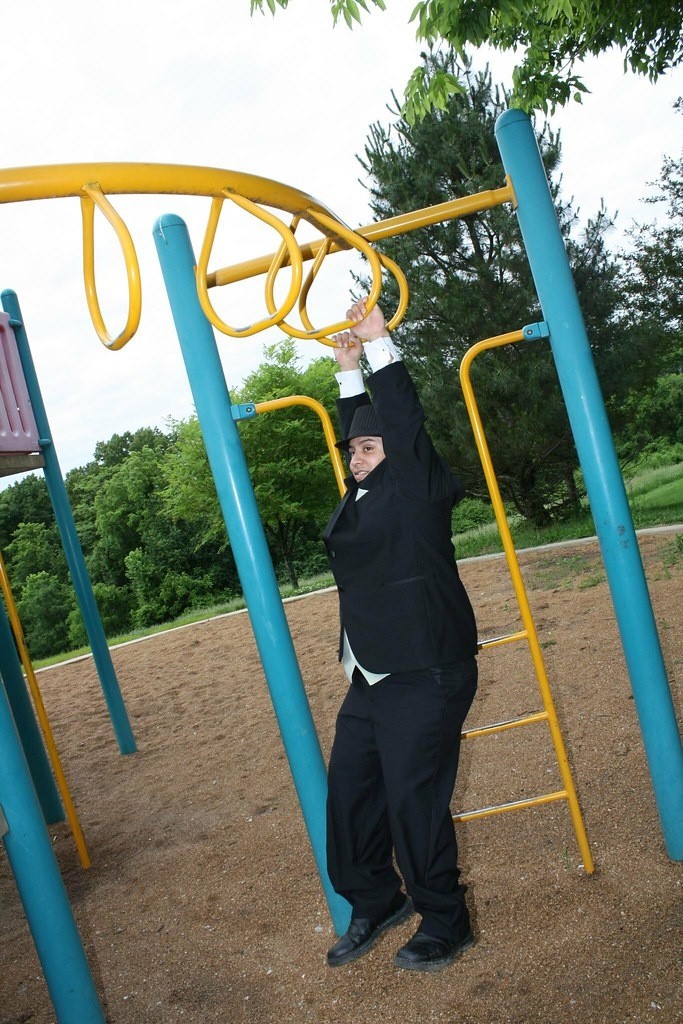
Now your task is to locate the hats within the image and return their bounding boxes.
[334,404,430,453]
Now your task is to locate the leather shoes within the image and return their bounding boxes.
[327,890,413,968]
[393,927,474,971]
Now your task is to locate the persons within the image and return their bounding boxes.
[323,296,479,971]
